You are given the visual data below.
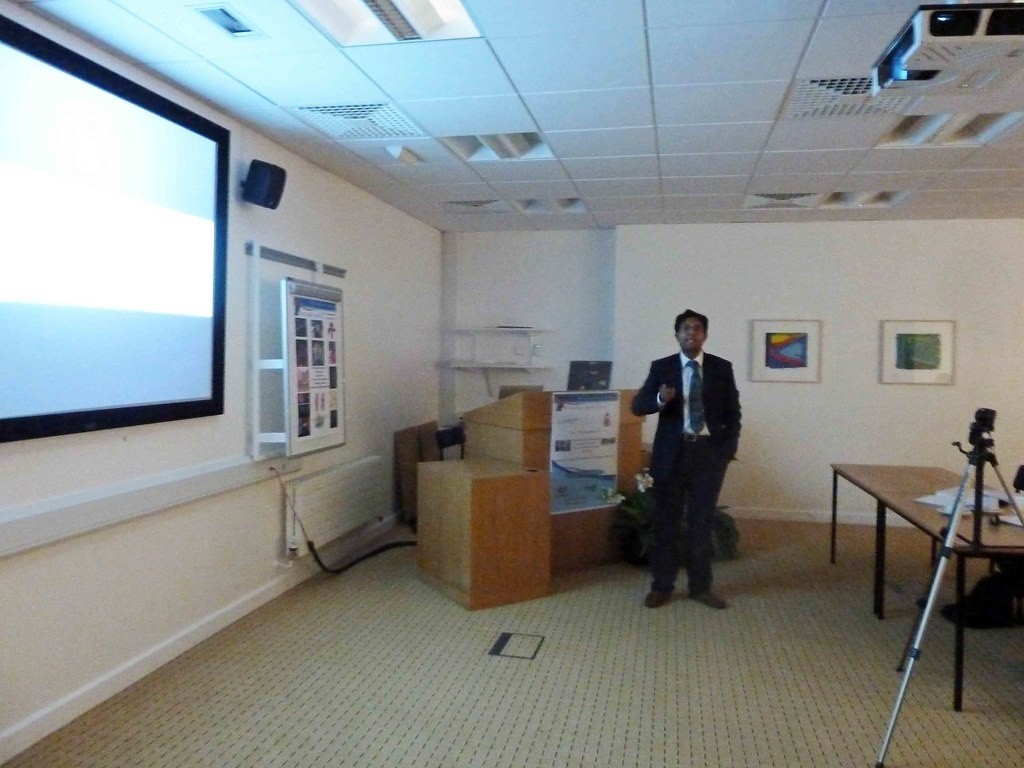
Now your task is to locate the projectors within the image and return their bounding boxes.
[871,3,1024,115]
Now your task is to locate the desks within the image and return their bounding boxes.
[830,463,1024,712]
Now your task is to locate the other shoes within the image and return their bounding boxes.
[646,585,669,607]
[690,591,725,608]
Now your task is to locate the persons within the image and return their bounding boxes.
[631,310,742,611]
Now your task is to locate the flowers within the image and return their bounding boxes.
[597,465,738,568]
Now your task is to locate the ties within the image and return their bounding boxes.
[687,359,706,435]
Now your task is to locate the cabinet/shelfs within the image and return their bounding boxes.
[450,329,553,370]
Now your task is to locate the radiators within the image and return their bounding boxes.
[286,455,384,566]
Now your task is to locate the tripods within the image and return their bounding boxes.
[879,425,1024,768]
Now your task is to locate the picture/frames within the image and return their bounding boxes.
[880,318,955,386]
[751,319,823,383]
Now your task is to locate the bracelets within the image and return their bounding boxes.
[659,395,664,403]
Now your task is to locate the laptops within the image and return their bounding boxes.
[567,361,612,392]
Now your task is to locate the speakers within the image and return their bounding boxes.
[242,160,287,209]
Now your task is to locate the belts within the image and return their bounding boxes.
[681,434,708,445]
